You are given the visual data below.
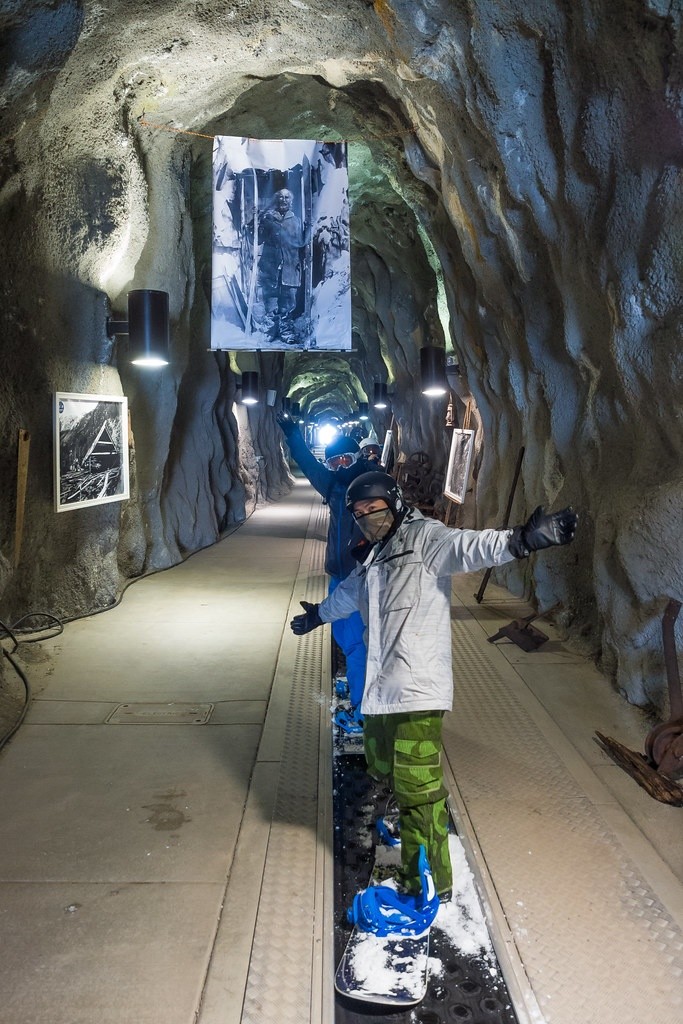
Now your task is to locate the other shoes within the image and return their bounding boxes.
[375,873,455,911]
[384,798,451,840]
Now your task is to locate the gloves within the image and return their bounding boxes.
[276,409,302,439]
[290,600,328,636]
[367,454,380,463]
[507,504,579,560]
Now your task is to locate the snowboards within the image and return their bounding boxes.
[332,792,432,1007]
[336,676,367,755]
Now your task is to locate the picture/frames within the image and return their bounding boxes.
[53,392,130,513]
[380,430,393,468]
[444,428,474,504]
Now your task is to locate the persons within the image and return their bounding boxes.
[276,409,387,737]
[244,190,305,347]
[291,468,581,906]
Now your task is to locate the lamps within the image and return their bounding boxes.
[337,411,360,428]
[106,289,170,366]
[421,346,448,395]
[282,398,290,417]
[236,371,259,404]
[374,383,396,409]
[291,403,321,430]
[359,402,370,420]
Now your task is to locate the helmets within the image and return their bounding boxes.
[344,471,405,521]
[321,435,364,479]
[358,437,381,452]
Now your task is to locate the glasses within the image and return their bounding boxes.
[324,449,363,472]
[360,445,379,454]
